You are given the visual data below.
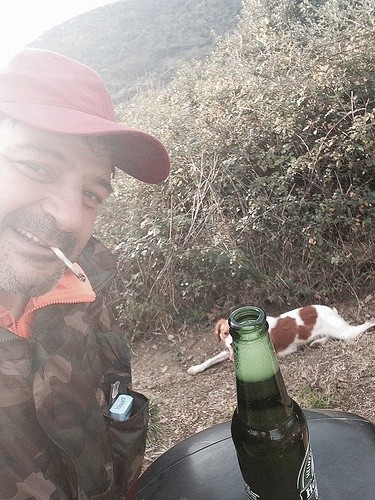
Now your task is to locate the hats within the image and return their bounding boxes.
[1,49,169,185]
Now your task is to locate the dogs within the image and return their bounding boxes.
[186,302,375,375]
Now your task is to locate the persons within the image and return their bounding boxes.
[0,49,150,499]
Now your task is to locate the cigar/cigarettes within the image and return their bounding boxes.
[51,245,87,283]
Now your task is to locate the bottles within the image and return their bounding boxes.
[224,304,321,500]
